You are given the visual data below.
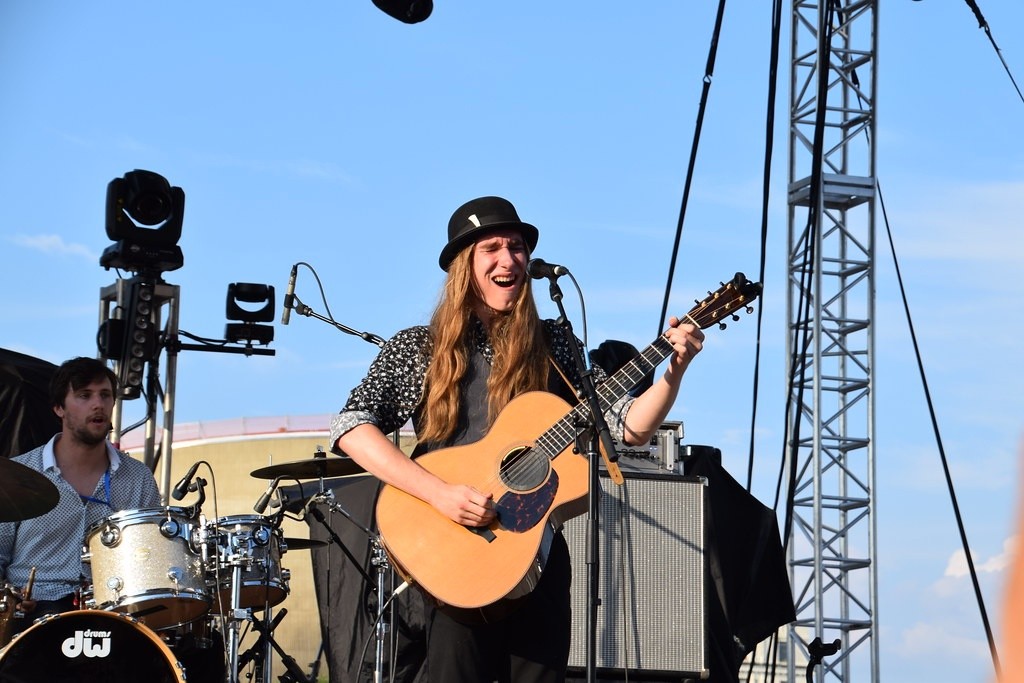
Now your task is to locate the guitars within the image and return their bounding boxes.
[373,272,763,616]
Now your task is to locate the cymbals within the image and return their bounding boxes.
[1,458,60,525]
[249,455,371,481]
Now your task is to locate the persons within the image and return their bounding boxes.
[0,353,208,638]
[330,195,706,683]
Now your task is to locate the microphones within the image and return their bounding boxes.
[526,258,568,279]
[282,265,297,325]
[254,478,279,514]
[171,462,200,501]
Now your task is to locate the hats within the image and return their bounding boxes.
[439,196,539,273]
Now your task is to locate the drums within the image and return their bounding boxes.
[204,515,290,614]
[0,610,189,683]
[84,506,212,631]
